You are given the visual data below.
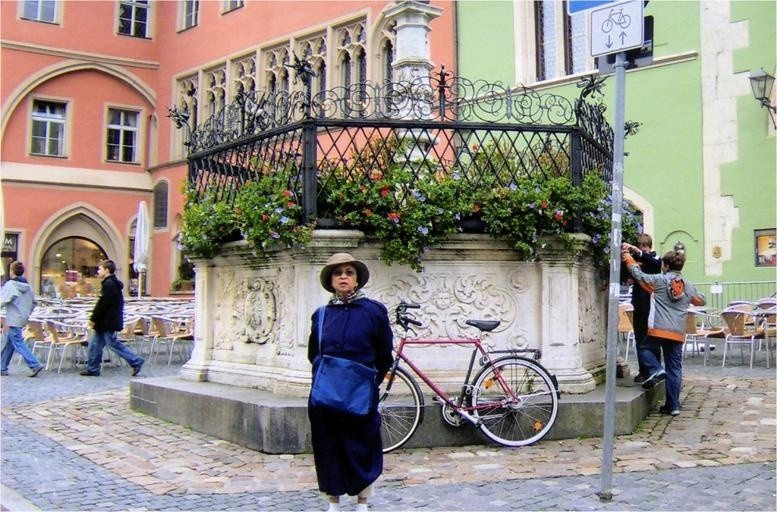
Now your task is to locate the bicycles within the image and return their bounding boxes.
[376,300,561,458]
[600,8,632,33]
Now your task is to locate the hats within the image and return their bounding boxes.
[319,252,369,294]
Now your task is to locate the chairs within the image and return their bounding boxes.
[3,295,195,373]
[618,296,776,370]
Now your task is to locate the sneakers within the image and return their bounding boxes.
[633,367,680,415]
[131,359,144,376]
[79,367,101,376]
[25,363,44,377]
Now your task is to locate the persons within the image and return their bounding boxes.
[307,253,393,512]
[621,234,662,383]
[80,260,145,376]
[0,261,43,377]
[622,250,706,415]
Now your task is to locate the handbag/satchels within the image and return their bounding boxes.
[310,354,379,417]
